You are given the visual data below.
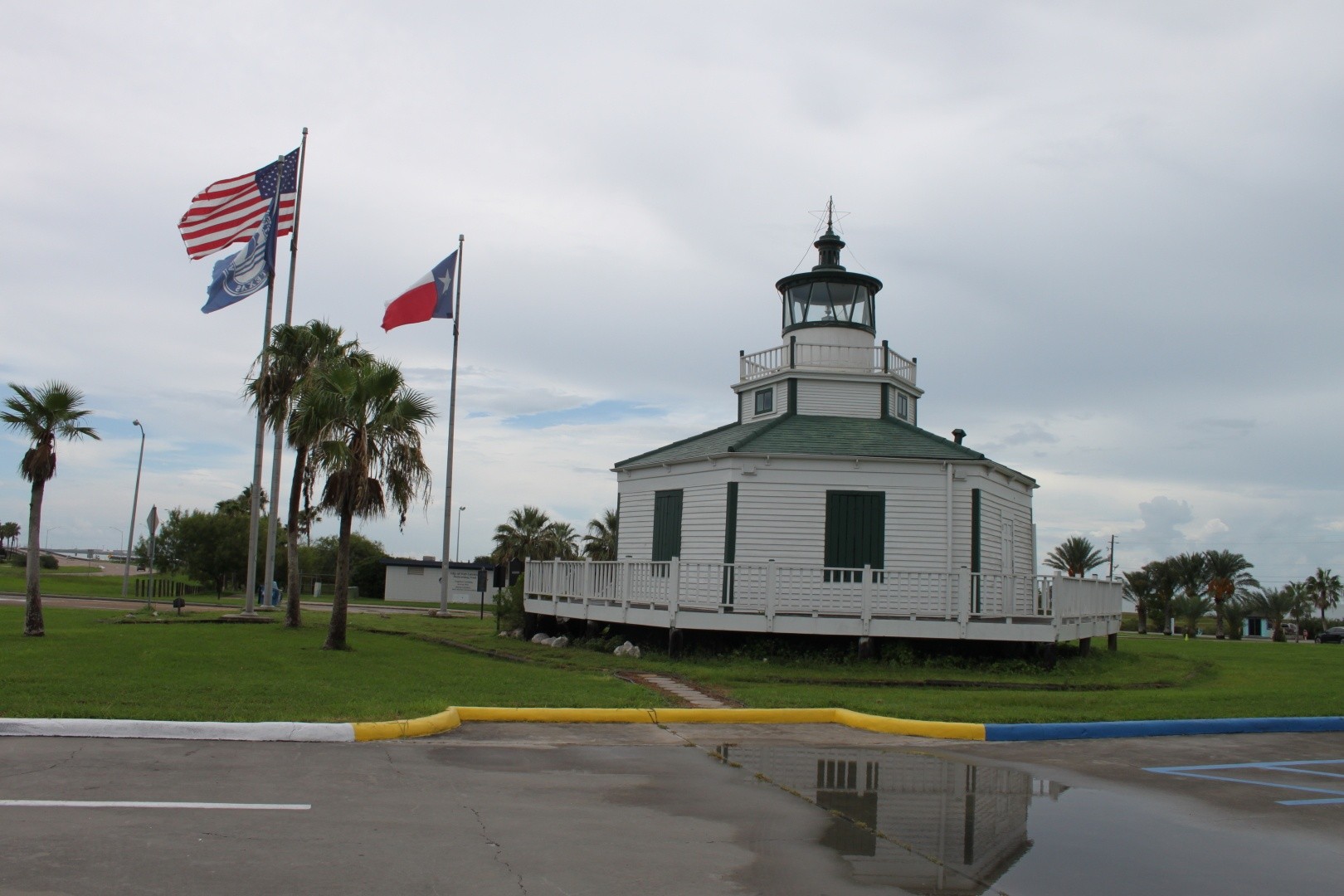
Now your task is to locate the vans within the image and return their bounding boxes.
[1281,623,1297,634]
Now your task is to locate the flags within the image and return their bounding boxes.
[178,147,300,264]
[201,165,282,314]
[380,249,457,333]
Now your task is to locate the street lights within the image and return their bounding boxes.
[45,526,60,554]
[110,526,123,563]
[457,505,466,561]
[122,419,146,596]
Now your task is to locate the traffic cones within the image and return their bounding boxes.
[1184,634,1189,641]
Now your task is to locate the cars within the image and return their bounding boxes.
[136,564,146,571]
[1315,626,1344,643]
